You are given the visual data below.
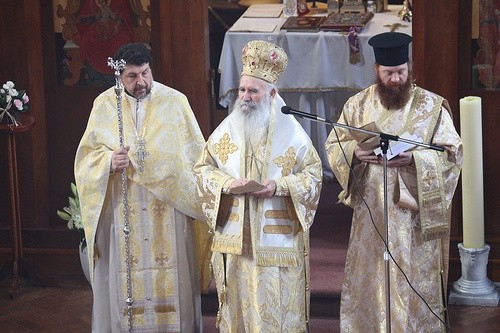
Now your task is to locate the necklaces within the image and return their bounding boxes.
[123,82,152,173]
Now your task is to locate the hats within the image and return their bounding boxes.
[368,32,413,66]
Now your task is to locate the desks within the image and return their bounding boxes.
[218,4,413,180]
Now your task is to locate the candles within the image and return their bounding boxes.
[459,96,484,247]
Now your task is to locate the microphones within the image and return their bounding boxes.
[281,106,330,123]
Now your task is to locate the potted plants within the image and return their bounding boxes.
[56,182,91,286]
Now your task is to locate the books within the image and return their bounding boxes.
[279,11,372,33]
[374,130,423,160]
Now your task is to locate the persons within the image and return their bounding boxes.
[74,42,205,332]
[192,40,324,332]
[324,32,464,333]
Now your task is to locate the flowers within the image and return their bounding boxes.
[0,81,29,127]
